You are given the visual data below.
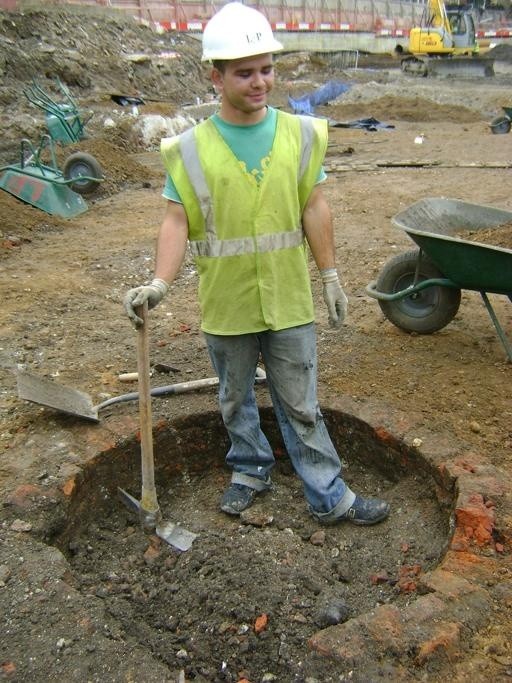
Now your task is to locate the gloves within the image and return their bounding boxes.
[320,267,348,329]
[124,278,169,330]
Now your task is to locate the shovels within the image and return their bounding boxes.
[16,367,267,423]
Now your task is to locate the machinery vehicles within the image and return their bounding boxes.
[395,0,497,82]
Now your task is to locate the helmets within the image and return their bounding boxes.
[200,3,284,63]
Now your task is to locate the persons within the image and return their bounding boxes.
[125,1,391,525]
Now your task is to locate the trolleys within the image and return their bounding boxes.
[23,74,94,148]
[366,198,512,361]
[0,133,108,222]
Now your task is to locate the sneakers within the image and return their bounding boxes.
[219,484,257,515]
[338,495,391,526]
